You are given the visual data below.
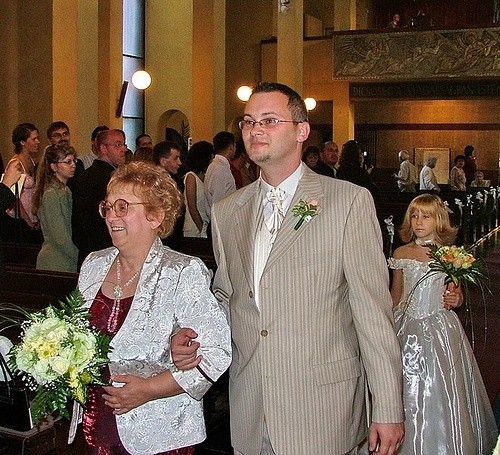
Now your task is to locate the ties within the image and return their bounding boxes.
[264,187,287,234]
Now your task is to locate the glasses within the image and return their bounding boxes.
[51,131,69,139]
[239,118,299,131]
[57,159,77,165]
[106,143,127,149]
[98,198,148,218]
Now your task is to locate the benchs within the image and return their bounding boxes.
[371,167,500,295]
[0,220,229,455]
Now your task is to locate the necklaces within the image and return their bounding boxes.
[107,256,141,332]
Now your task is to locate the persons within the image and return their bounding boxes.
[171,82,406,455]
[450,145,491,233]
[386,14,408,28]
[78,126,255,251]
[387,194,497,455]
[68,159,232,455]
[0,122,78,273]
[301,139,440,209]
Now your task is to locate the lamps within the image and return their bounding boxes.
[237,85,252,101]
[304,68,317,111]
[132,67,152,91]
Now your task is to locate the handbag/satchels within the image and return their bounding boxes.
[0,352,33,431]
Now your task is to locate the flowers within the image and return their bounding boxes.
[399,243,497,379]
[0,279,145,427]
[291,198,320,231]
